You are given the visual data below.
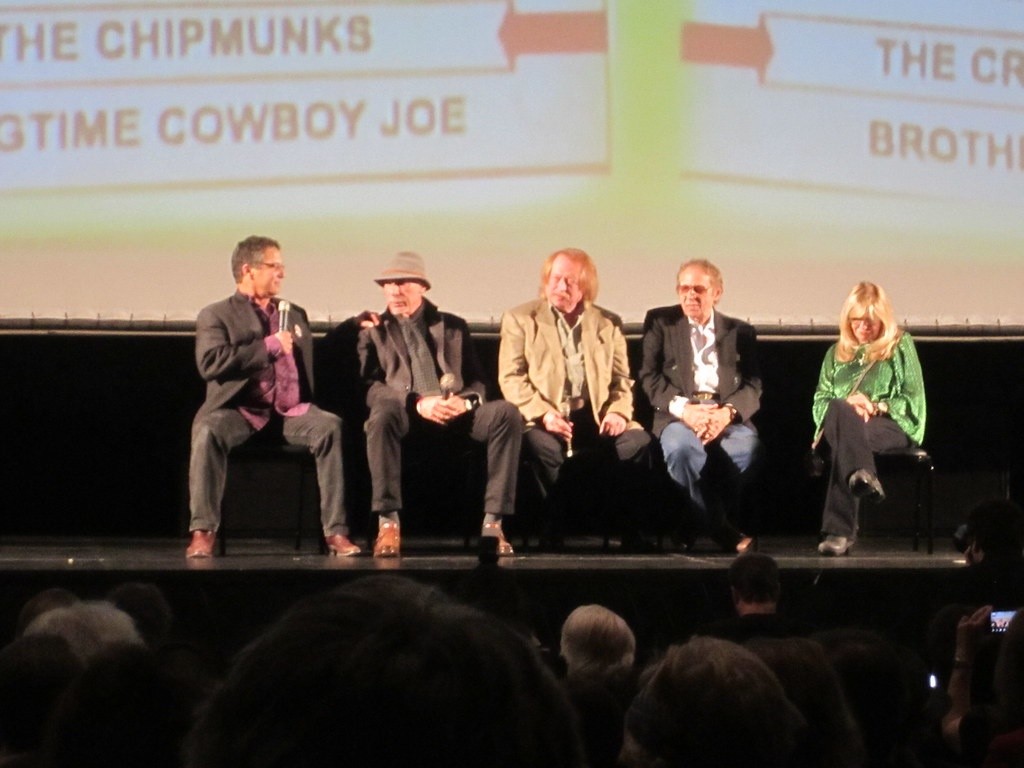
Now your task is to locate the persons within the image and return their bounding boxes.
[0,571,1024,768]
[352,252,525,555]
[809,281,926,555]
[498,248,678,550]
[183,234,382,555]
[638,258,767,555]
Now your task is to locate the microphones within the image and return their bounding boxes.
[558,402,573,458]
[278,301,289,333]
[441,373,456,400]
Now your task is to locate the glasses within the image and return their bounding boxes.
[249,261,285,271]
[677,284,716,295]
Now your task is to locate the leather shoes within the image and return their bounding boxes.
[818,532,855,557]
[849,468,885,505]
[325,533,361,556]
[483,520,514,556]
[373,523,402,557]
[185,528,220,559]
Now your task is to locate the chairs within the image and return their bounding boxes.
[219,410,931,556]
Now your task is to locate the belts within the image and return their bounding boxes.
[690,391,722,400]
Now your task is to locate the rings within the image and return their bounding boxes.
[694,428,699,432]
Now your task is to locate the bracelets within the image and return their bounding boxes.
[724,404,738,420]
[464,399,473,411]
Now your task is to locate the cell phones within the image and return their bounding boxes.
[986,609,1016,636]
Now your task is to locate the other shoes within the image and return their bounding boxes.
[621,529,650,554]
[537,527,566,553]
[670,523,698,552]
[713,520,753,556]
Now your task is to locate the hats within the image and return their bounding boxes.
[374,251,433,289]
